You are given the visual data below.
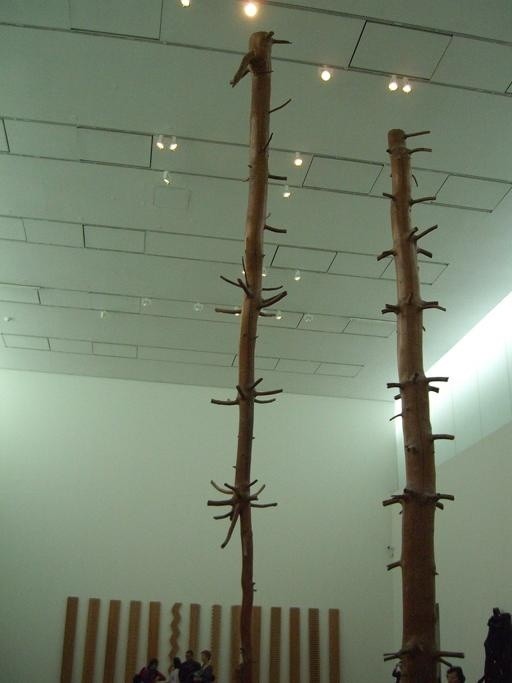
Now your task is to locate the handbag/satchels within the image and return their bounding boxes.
[478,607,512,683]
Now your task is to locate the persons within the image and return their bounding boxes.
[199,650,216,683]
[178,650,202,682]
[166,656,181,683]
[446,666,464,683]
[135,657,166,683]
[392,649,403,682]
